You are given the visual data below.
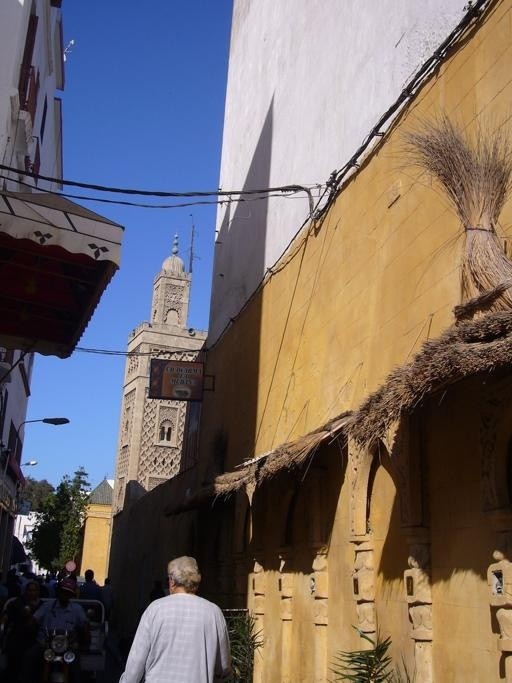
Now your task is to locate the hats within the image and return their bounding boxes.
[61,579,76,593]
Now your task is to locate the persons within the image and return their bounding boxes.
[0,566,112,683]
[119,556,232,683]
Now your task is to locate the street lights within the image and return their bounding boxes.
[13,417,70,458]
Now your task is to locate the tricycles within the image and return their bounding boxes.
[0,596,109,683]
